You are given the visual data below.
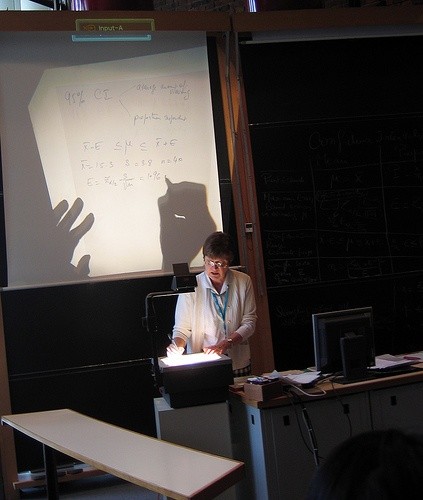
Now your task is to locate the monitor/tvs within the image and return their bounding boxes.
[309,306,377,384]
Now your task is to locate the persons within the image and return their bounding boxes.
[167,232,257,377]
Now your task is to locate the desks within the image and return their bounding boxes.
[0,409,248,500]
[227,350,423,500]
[152,397,237,500]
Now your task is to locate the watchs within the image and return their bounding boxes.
[227,338,232,348]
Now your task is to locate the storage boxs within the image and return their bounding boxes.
[243,380,283,402]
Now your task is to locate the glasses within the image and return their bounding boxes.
[206,259,226,267]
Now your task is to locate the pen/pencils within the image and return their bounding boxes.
[167,333,180,355]
[404,357,421,360]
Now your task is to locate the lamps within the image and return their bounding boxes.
[139,263,198,399]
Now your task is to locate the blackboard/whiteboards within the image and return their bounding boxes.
[220,107,423,295]
